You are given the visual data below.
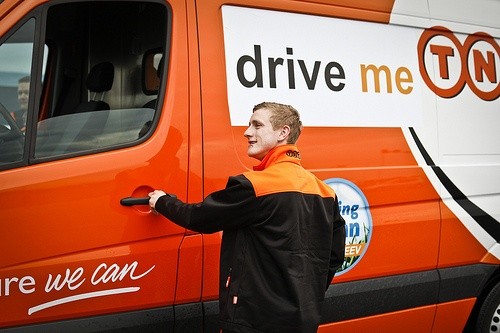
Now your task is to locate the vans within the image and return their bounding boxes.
[0,1,500,333]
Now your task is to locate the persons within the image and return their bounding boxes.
[150,102,345,333]
[10,76,33,132]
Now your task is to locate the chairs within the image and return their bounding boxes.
[38,61,115,159]
